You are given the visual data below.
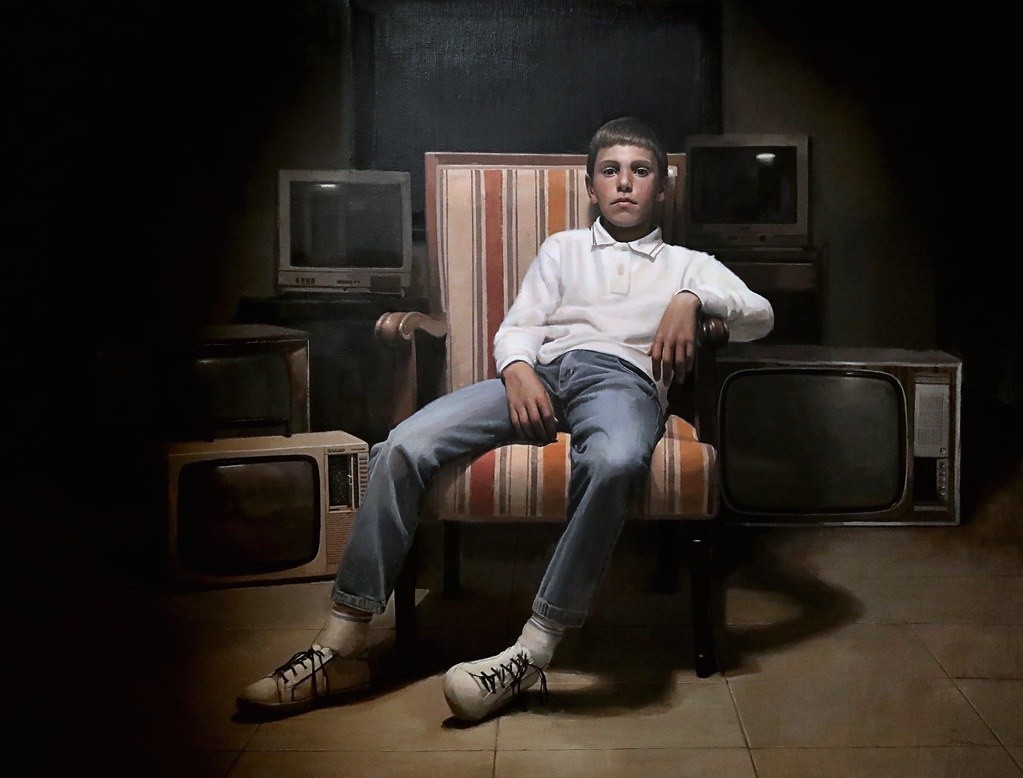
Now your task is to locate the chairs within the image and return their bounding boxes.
[373,152,730,677]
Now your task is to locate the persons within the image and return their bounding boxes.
[237,116,774,723]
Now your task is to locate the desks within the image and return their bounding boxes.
[712,250,825,295]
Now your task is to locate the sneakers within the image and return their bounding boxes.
[443,643,550,721]
[237,643,374,717]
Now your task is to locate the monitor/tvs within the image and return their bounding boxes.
[165,322,371,588]
[277,169,413,293]
[692,341,962,528]
[685,132,810,248]
